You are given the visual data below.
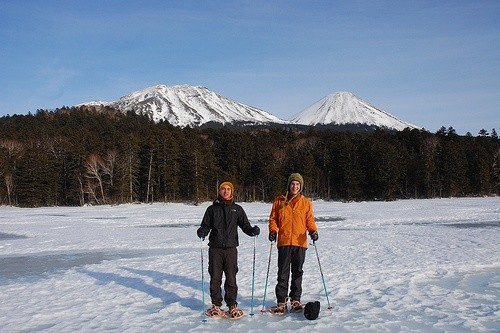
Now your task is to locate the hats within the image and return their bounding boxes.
[280,173,304,208]
[217,176,237,195]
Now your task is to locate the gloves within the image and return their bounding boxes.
[309,230,318,241]
[197,227,206,241]
[252,225,260,235]
[269,230,277,242]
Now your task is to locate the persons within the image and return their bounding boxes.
[196,182,260,317]
[268,172,319,316]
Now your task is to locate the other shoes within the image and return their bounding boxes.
[273,297,292,315]
[211,306,220,317]
[229,308,243,318]
[291,301,302,311]
